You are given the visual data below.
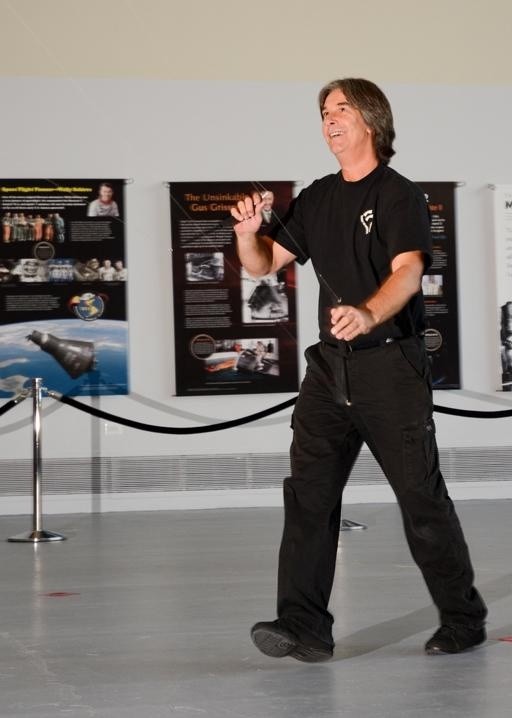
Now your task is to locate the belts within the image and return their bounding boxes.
[318,317,424,353]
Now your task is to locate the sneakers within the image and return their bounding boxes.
[249,614,335,665]
[423,618,489,656]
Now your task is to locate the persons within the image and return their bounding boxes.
[113,260,128,282]
[87,182,119,218]
[256,189,275,226]
[226,74,494,663]
[99,259,115,280]
[47,258,74,282]
[88,257,100,283]
[3,209,65,244]
